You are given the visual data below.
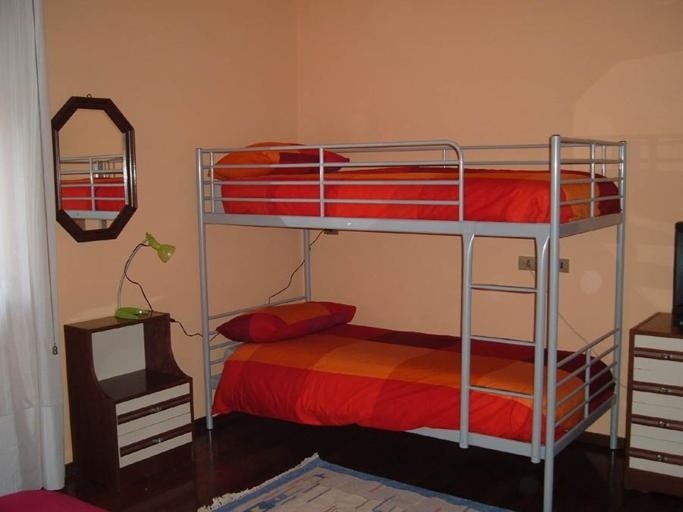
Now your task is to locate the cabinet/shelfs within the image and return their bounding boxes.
[624,313,683,497]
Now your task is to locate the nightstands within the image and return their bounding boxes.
[64,309,196,491]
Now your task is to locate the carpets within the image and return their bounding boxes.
[196,451,513,512]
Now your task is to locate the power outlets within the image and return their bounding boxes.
[518,256,537,270]
[325,230,338,234]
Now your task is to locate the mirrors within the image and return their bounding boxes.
[51,96,138,242]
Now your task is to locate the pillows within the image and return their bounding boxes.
[216,301,357,344]
[208,142,350,181]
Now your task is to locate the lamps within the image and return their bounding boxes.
[115,232,176,319]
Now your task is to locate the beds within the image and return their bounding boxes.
[196,134,627,512]
[60,155,125,229]
